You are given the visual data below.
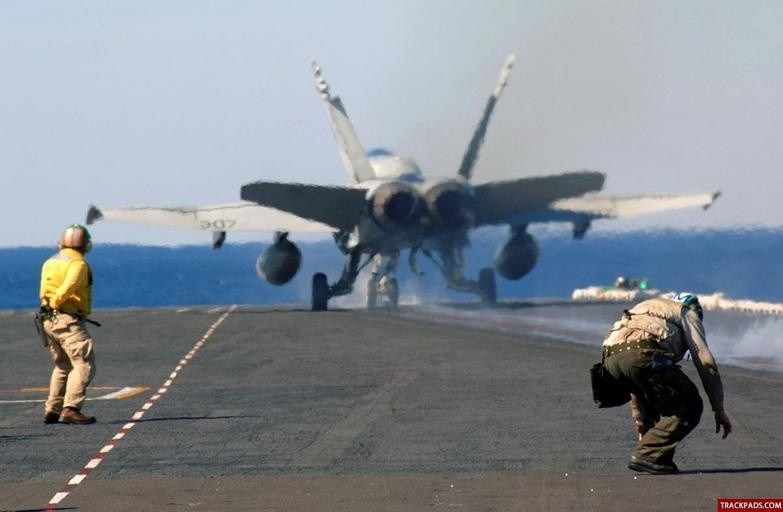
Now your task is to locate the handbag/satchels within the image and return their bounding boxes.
[588,353,631,409]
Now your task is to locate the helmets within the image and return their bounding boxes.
[672,291,703,319]
[62,222,91,250]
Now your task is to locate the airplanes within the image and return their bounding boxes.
[81,58,722,314]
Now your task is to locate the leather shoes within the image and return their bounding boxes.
[43,411,59,423]
[627,453,679,473]
[58,408,97,424]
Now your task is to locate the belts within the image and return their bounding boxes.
[605,341,654,356]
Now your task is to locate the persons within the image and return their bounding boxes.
[600,290,731,473]
[38,223,99,425]
